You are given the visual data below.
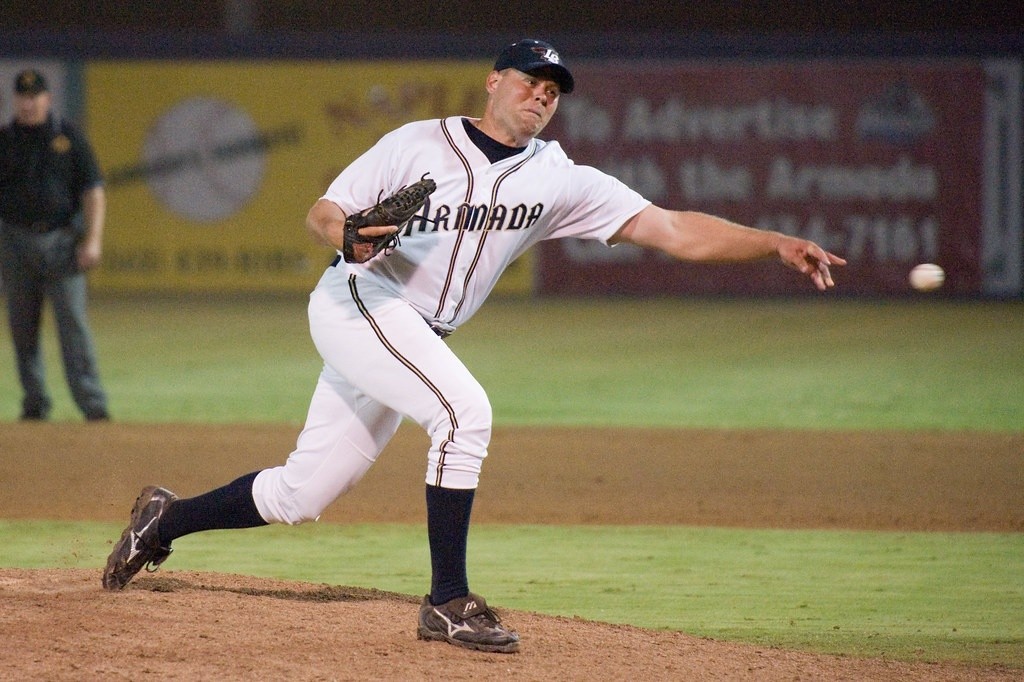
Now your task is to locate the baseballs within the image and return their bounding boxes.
[908,263,946,292]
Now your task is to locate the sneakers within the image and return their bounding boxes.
[101,485,177,590]
[418,592,521,653]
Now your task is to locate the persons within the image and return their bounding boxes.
[101,38,848,655]
[0,65,111,424]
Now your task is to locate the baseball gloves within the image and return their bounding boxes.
[342,170,439,264]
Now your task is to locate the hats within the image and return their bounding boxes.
[494,38,575,95]
[13,69,49,97]
